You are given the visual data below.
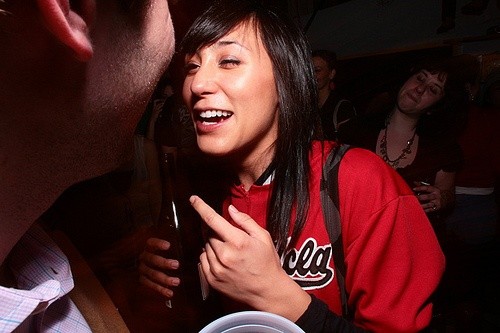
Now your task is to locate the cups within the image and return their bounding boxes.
[197,311,308,333]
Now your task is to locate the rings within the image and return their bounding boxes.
[431,202,436,209]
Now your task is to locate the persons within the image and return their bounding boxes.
[0,0,177,333]
[147,46,466,213]
[137,1,446,333]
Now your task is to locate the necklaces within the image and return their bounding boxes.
[380,128,417,166]
